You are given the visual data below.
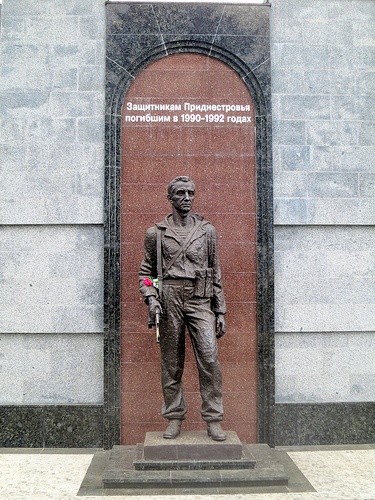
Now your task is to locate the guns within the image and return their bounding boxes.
[154,306,161,343]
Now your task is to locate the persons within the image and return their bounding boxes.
[137,175,228,441]
